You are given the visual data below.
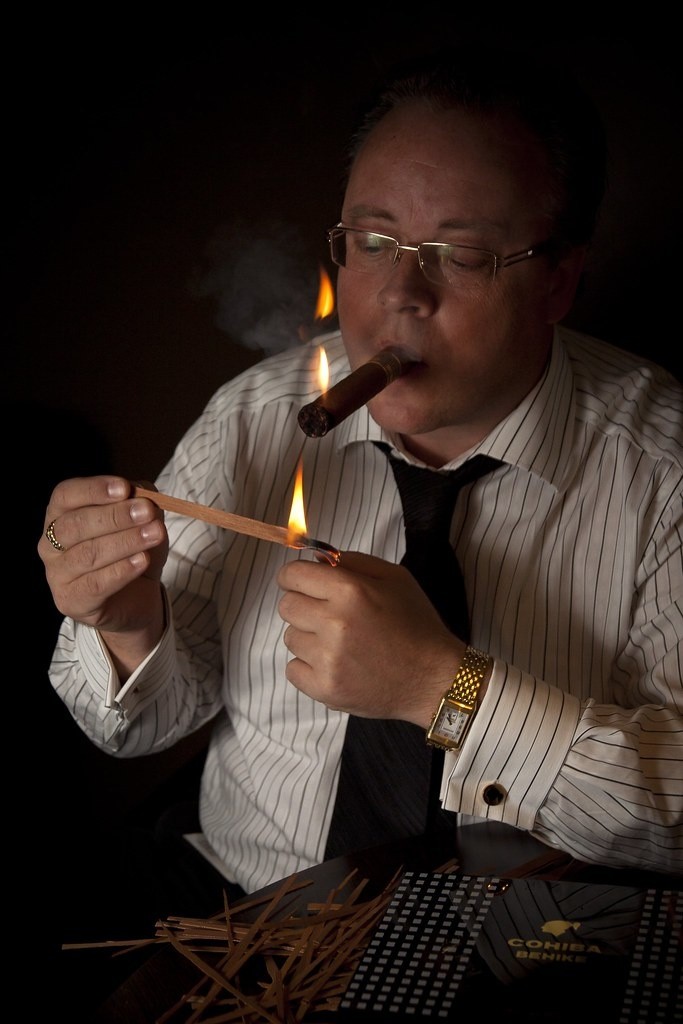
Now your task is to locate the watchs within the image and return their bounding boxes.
[426,644,492,754]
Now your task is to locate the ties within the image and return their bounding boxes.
[323,440,509,863]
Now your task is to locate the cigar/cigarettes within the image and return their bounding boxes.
[297,345,410,439]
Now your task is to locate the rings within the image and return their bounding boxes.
[47,520,68,553]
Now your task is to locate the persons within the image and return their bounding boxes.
[37,80,682,919]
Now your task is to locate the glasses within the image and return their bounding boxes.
[325,222,569,291]
